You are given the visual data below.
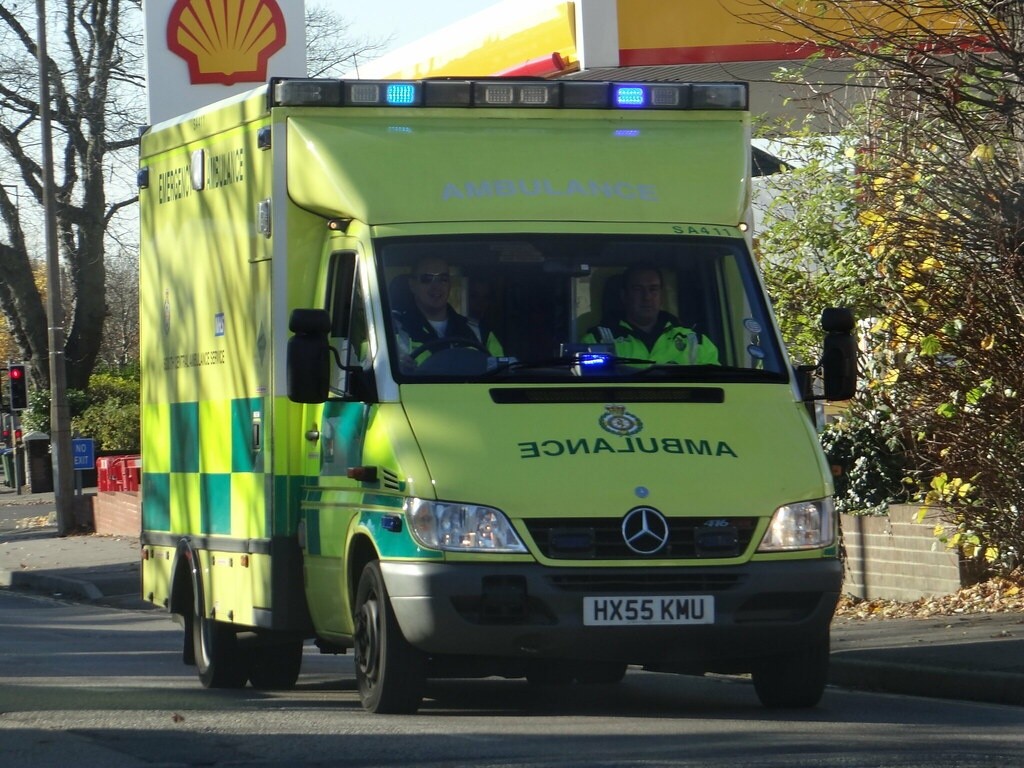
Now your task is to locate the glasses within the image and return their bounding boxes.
[419,274,450,286]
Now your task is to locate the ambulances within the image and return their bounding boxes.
[137,74,859,716]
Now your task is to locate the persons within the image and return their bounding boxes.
[581,262,721,366]
[358,250,507,372]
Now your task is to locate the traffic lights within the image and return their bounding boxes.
[8,364,28,411]
